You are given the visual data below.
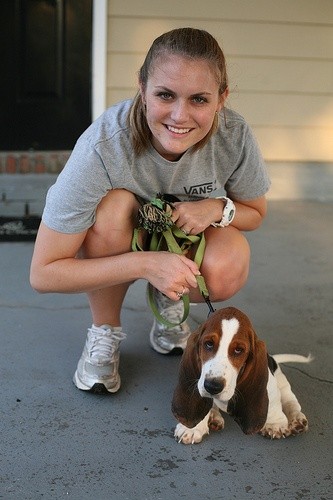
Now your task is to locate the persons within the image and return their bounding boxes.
[27,27,270,393]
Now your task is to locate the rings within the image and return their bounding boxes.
[177,286,185,297]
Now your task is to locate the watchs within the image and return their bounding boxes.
[211,196,236,228]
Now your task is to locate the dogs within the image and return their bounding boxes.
[170,306,308,444]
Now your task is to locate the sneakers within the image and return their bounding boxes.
[72,323,126,395]
[149,287,192,356]
